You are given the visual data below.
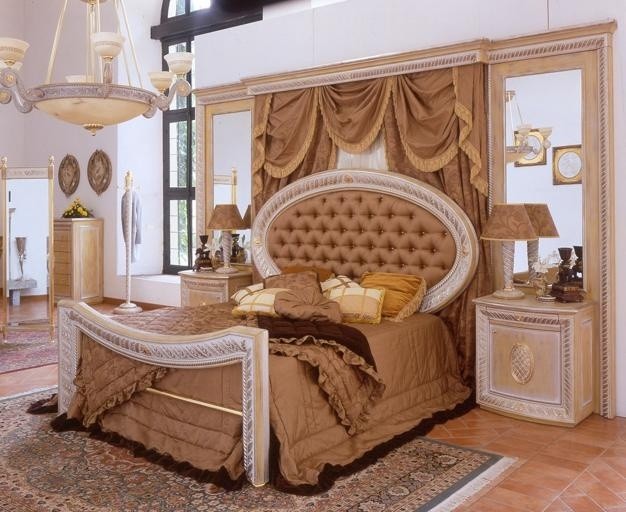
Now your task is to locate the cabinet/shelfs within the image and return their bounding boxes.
[51,218,103,306]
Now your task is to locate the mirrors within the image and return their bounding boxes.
[204,97,255,268]
[488,49,599,302]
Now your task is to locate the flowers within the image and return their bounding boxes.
[61,197,96,218]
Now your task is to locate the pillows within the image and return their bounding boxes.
[229,264,428,324]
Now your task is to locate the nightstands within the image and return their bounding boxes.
[472,290,598,429]
[178,268,253,306]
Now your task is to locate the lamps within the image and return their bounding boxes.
[504,89,554,167]
[480,203,538,301]
[241,204,251,261]
[0,1,194,137]
[523,202,561,286]
[206,204,247,274]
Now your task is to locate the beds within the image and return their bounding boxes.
[54,167,482,490]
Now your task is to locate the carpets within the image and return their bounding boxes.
[1,384,526,512]
[0,324,56,374]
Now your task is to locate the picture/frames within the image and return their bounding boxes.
[552,144,582,186]
[513,130,548,167]
